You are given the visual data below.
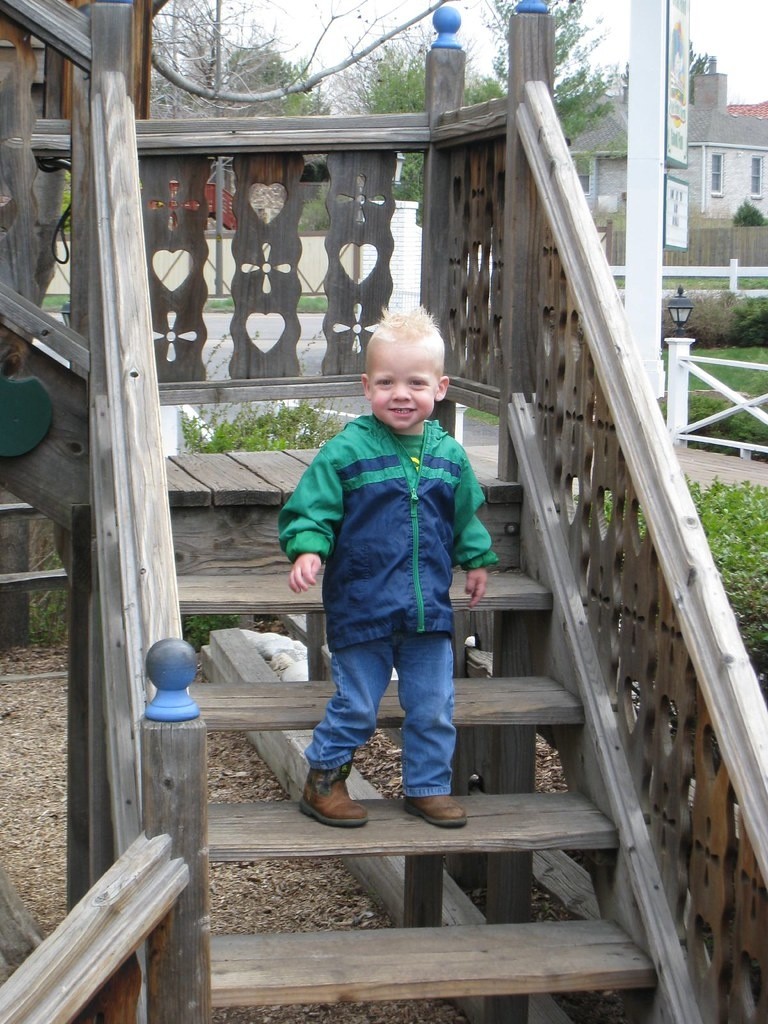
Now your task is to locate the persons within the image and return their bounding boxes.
[276,303,500,828]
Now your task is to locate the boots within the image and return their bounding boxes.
[300,761,368,826]
[404,794,466,826]
